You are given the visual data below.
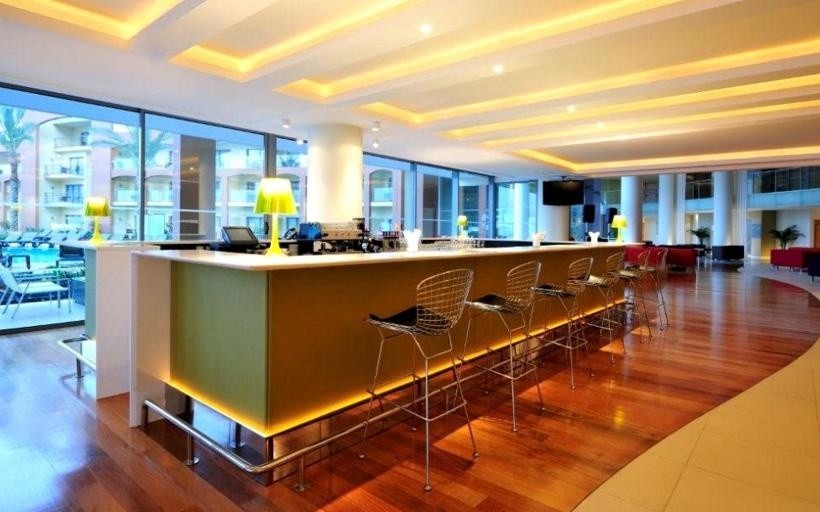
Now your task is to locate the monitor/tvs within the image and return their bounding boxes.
[542,180,584,205]
[222,225,259,247]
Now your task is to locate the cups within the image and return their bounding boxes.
[533,236,542,246]
[397,229,485,253]
[591,236,599,244]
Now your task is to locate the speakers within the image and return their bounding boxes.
[583,204,595,223]
[605,208,617,224]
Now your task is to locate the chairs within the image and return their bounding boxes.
[0,220,127,319]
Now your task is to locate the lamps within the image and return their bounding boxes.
[611,215,628,242]
[252,178,297,256]
[456,215,467,239]
[84,196,111,242]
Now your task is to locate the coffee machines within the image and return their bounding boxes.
[298,220,370,254]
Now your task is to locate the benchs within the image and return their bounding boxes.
[770,247,820,282]
[626,245,745,272]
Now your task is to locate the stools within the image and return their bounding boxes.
[519,253,670,390]
[449,260,547,434]
[358,268,481,491]
